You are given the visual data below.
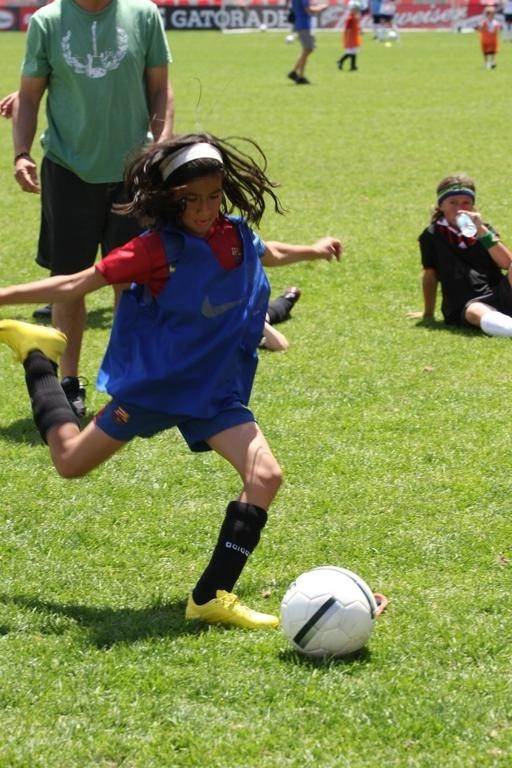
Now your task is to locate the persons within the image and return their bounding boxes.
[12,1,175,418]
[0,90,52,318]
[288,0,512,84]
[0,134,342,630]
[407,176,512,337]
[257,286,301,351]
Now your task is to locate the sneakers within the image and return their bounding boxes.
[32,304,54,318]
[288,70,315,85]
[0,317,68,366]
[184,587,280,631]
[280,286,301,321]
[61,376,88,418]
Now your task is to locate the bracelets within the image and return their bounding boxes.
[15,152,29,164]
[479,231,500,250]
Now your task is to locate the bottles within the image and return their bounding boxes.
[455,212,477,238]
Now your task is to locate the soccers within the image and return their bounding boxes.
[388,30,397,40]
[258,23,268,33]
[286,35,295,44]
[278,565,376,657]
